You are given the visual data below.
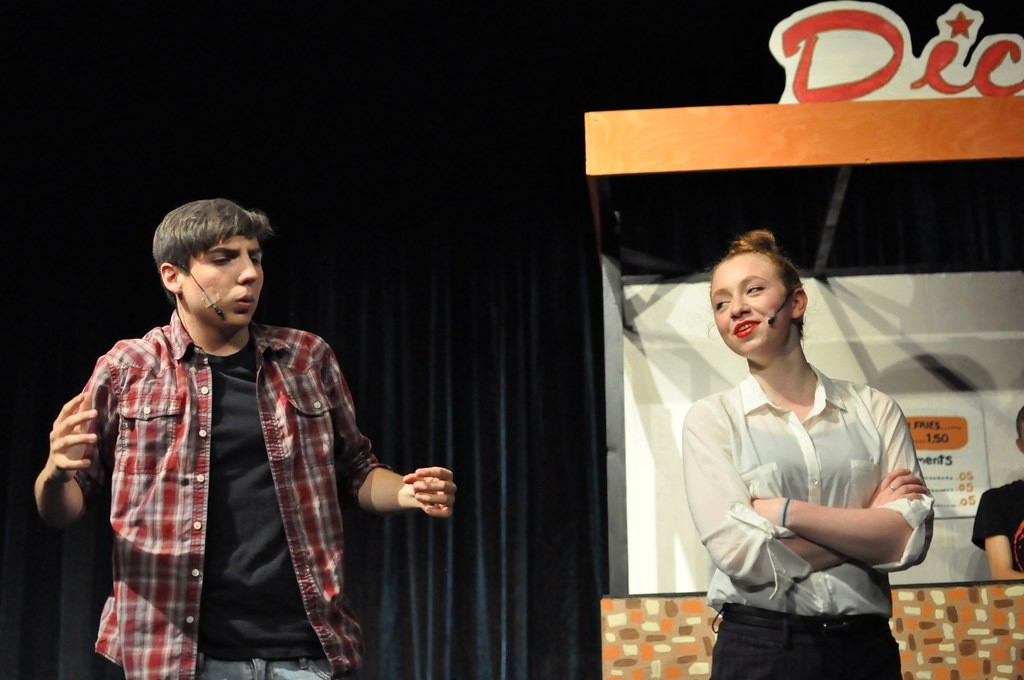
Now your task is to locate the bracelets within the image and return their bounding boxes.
[781,496,792,528]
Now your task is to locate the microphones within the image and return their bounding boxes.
[768,291,792,325]
[177,263,223,317]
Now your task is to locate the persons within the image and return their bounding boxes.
[972,406,1024,581]
[34,198,456,680]
[682,230,934,680]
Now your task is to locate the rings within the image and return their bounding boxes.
[889,484,896,492]
